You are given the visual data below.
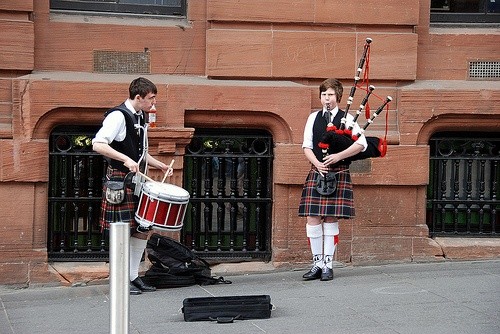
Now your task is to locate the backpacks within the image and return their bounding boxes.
[144,234,212,277]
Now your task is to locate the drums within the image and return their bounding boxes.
[134,181,190,232]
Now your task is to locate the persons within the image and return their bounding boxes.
[92,77,173,295]
[298,78,368,281]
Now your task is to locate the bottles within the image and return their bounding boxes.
[148,104,156,127]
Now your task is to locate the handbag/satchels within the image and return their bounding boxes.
[180,295,274,323]
[105,180,125,204]
[315,171,337,196]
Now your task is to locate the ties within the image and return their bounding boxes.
[324,111,333,123]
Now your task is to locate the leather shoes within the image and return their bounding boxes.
[132,276,157,291]
[320,265,333,281]
[303,266,321,279]
[129,281,143,295]
